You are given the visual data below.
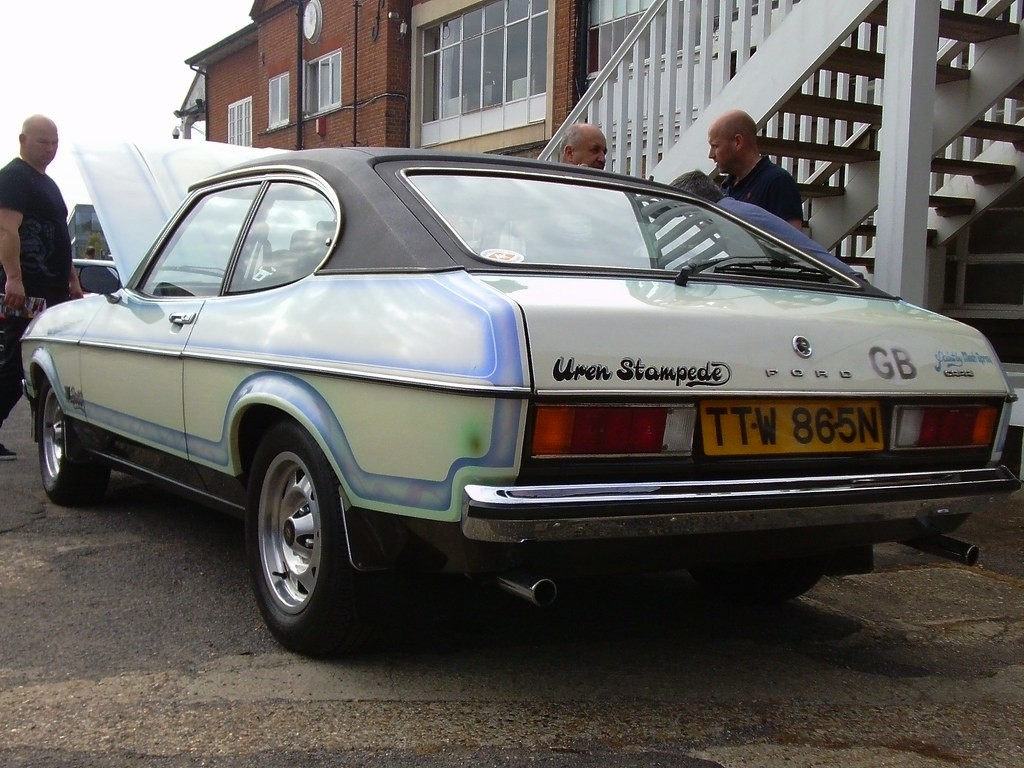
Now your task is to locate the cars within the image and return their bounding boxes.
[72,259,119,295]
[18,129,1023,663]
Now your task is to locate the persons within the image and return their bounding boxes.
[669,169,869,284]
[708,109,803,230]
[558,123,608,171]
[0,114,85,459]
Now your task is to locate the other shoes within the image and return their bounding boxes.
[0,443,17,460]
